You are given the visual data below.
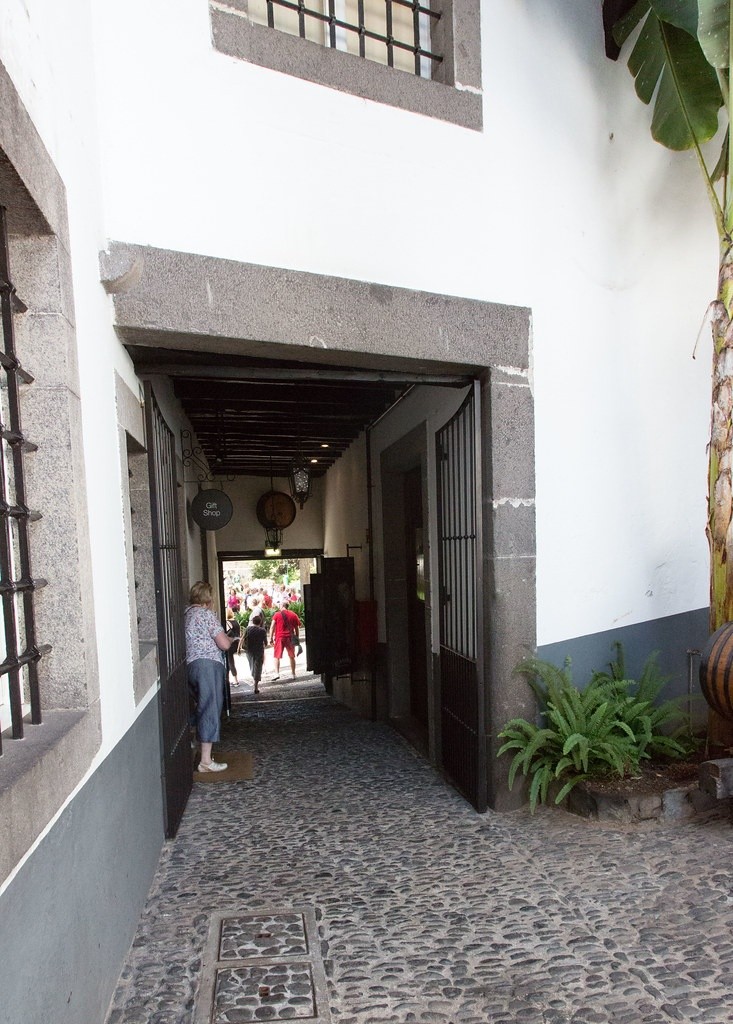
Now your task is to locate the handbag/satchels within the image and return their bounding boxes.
[242,638,252,650]
[291,636,300,646]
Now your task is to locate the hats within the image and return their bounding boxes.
[297,645,303,657]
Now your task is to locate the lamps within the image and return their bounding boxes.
[263,454,284,556]
[287,441,314,510]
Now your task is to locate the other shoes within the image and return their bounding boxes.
[254,689,260,694]
[292,674,297,680]
[271,674,280,681]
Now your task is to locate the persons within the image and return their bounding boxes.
[224,580,302,694]
[183,581,233,773]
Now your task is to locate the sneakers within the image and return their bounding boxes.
[230,682,240,687]
[197,760,228,773]
